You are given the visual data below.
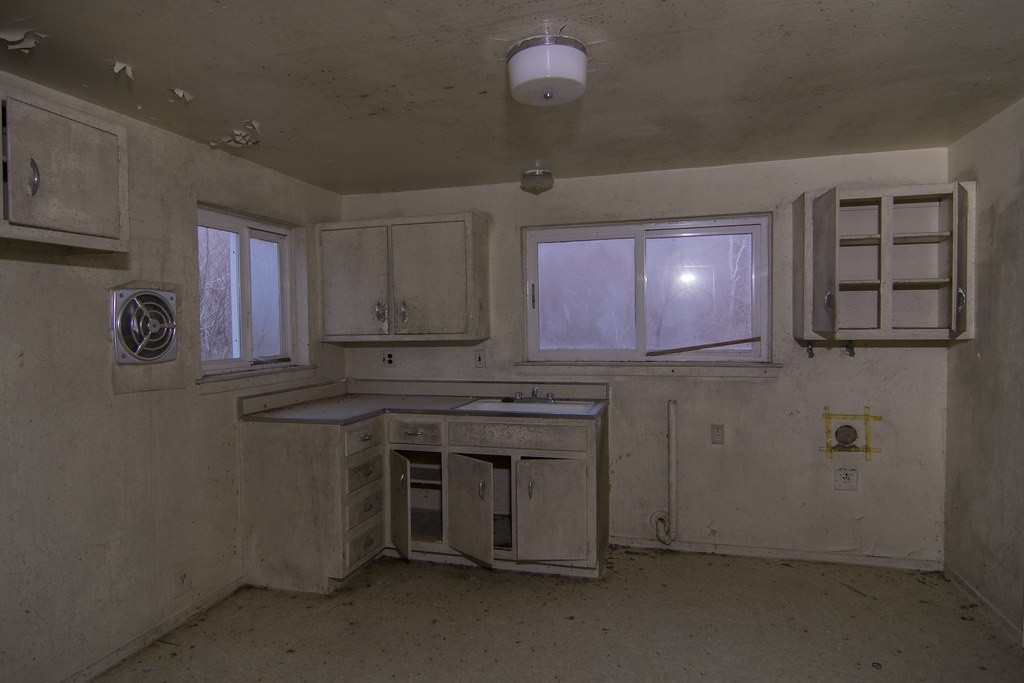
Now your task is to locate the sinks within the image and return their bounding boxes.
[453,398,598,416]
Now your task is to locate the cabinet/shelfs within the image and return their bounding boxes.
[316,212,490,343]
[237,420,388,593]
[0,83,130,254]
[443,412,610,570]
[385,419,446,560]
[794,180,976,350]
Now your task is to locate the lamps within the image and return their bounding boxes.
[504,35,590,107]
[522,169,552,193]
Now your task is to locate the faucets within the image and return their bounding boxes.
[531,386,538,403]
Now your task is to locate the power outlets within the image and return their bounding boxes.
[711,424,725,445]
[834,469,859,490]
